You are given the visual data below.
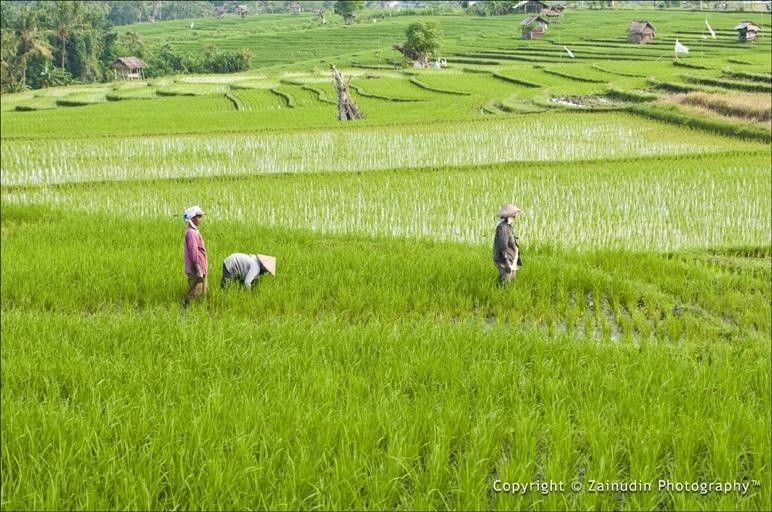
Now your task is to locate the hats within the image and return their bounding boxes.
[494,204,521,216]
[256,254,276,276]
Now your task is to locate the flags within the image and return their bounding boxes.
[675,41,689,54]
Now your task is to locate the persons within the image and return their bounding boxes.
[221,253,276,291]
[182,206,207,310]
[493,204,523,286]
